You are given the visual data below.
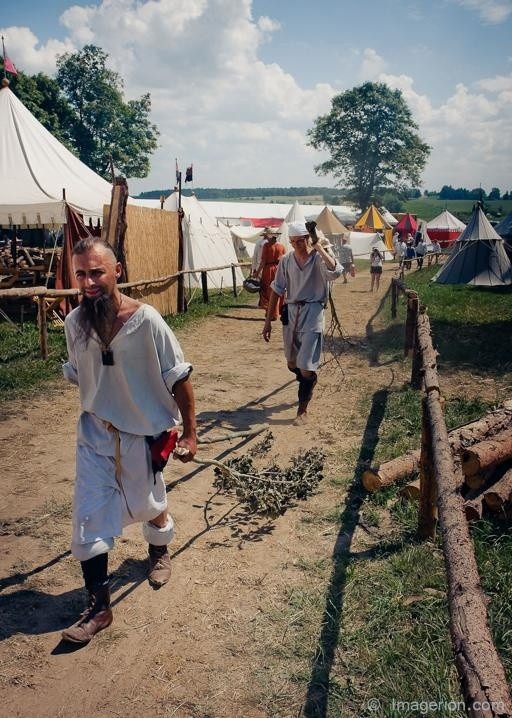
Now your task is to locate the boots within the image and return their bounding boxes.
[59,576,113,644]
[143,513,176,587]
[288,366,317,428]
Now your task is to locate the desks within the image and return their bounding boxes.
[20,265,56,286]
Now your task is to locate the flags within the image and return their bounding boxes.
[3,42,18,76]
[175,160,182,185]
[184,166,193,183]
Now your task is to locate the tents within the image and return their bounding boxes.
[491,208,512,266]
[424,199,512,288]
[425,208,468,250]
[345,229,396,262]
[313,205,349,249]
[273,197,309,255]
[352,201,394,253]
[154,185,246,291]
[392,212,419,241]
[0,76,166,230]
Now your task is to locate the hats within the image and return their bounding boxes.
[258,225,283,236]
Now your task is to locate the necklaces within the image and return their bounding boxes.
[79,296,124,367]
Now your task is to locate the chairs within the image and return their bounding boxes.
[17,249,35,266]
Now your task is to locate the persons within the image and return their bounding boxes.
[251,230,273,278]
[391,231,441,271]
[59,236,200,645]
[368,247,383,293]
[254,232,287,322]
[260,219,341,430]
[334,238,353,284]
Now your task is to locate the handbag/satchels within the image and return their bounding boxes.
[349,262,357,277]
[151,428,180,473]
[278,304,289,325]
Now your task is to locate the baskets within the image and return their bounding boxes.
[243,274,262,293]
